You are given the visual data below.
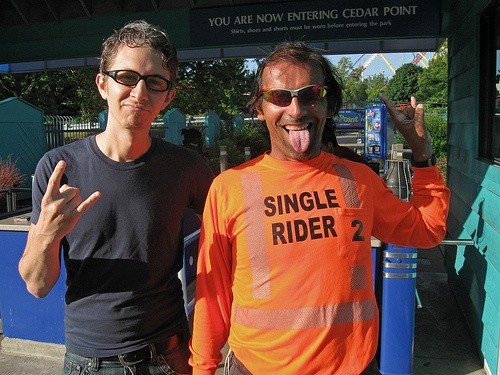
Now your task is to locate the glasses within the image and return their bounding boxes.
[260,84,329,107]
[103,70,173,92]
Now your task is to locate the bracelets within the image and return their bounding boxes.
[410,152,436,168]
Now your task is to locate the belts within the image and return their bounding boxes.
[100,326,190,362]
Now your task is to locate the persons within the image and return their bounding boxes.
[181,128,212,166]
[321,118,368,164]
[188,41,452,375]
[18,20,216,375]
